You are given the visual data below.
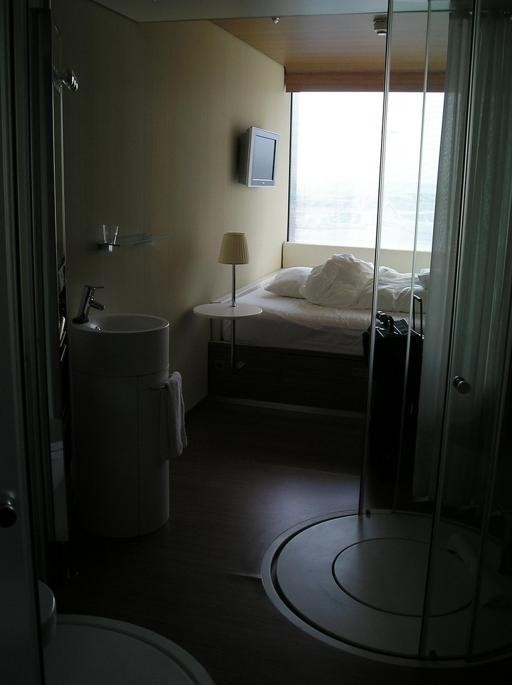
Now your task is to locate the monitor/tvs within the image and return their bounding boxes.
[239,126,280,189]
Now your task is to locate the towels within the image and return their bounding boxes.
[161,369,188,457]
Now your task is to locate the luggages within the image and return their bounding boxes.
[360,295,426,498]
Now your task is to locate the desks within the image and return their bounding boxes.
[191,300,265,370]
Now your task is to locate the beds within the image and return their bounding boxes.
[208,238,432,423]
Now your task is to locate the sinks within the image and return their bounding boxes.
[69,313,170,376]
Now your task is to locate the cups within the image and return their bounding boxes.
[102,224,119,244]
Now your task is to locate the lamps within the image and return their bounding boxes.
[218,233,253,308]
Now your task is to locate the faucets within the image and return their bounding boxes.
[74,284,105,323]
